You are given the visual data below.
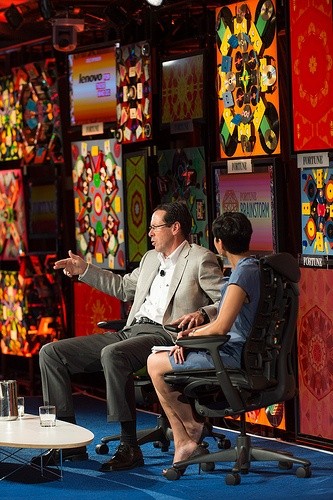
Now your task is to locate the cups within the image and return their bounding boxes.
[18,397,25,417]
[39,406,56,427]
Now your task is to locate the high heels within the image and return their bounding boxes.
[196,423,217,446]
[163,445,203,475]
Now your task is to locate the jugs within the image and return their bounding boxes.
[0,380,18,421]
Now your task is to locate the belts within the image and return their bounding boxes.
[134,317,155,324]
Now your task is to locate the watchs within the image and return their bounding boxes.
[200,308,209,325]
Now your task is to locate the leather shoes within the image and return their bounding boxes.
[99,444,144,472]
[31,446,88,467]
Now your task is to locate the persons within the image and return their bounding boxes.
[29,203,228,472]
[147,212,262,481]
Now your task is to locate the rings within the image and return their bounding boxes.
[177,350,180,353]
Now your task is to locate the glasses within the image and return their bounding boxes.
[149,223,170,230]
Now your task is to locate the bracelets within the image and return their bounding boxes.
[191,329,197,336]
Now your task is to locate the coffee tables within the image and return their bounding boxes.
[0,413,94,481]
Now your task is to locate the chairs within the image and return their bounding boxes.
[94,253,312,485]
